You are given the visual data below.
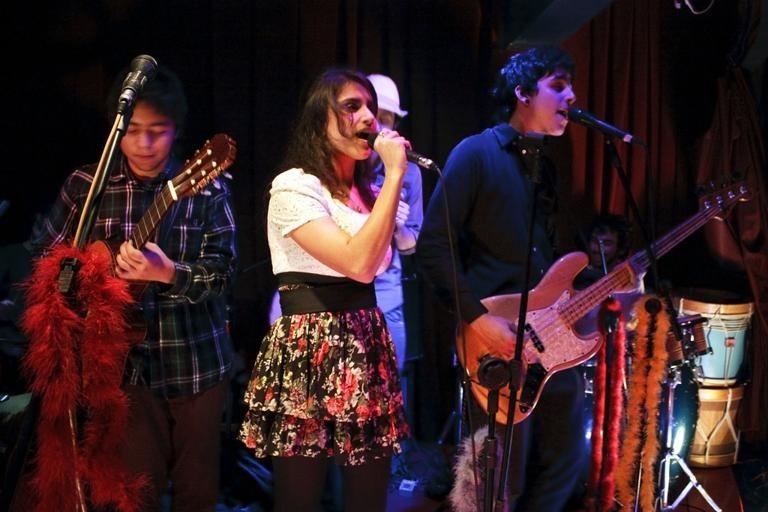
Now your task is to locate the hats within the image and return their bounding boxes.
[366,74,408,118]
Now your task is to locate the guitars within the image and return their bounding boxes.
[87,130,238,347]
[451,168,757,428]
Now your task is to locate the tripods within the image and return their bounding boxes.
[616,145,727,512]
[484,138,545,511]
[38,105,144,510]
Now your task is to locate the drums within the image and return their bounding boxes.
[577,358,701,490]
[668,287,756,387]
[664,311,713,365]
[689,380,752,468]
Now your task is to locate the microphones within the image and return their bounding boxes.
[119,53,159,103]
[366,133,436,172]
[566,107,633,142]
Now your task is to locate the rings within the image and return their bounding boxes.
[380,131,388,140]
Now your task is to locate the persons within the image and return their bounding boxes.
[243,72,410,511]
[266,76,425,509]
[32,69,235,512]
[417,46,647,511]
[696,133,768,511]
[577,214,646,338]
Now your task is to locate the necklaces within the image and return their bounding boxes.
[337,186,369,212]
